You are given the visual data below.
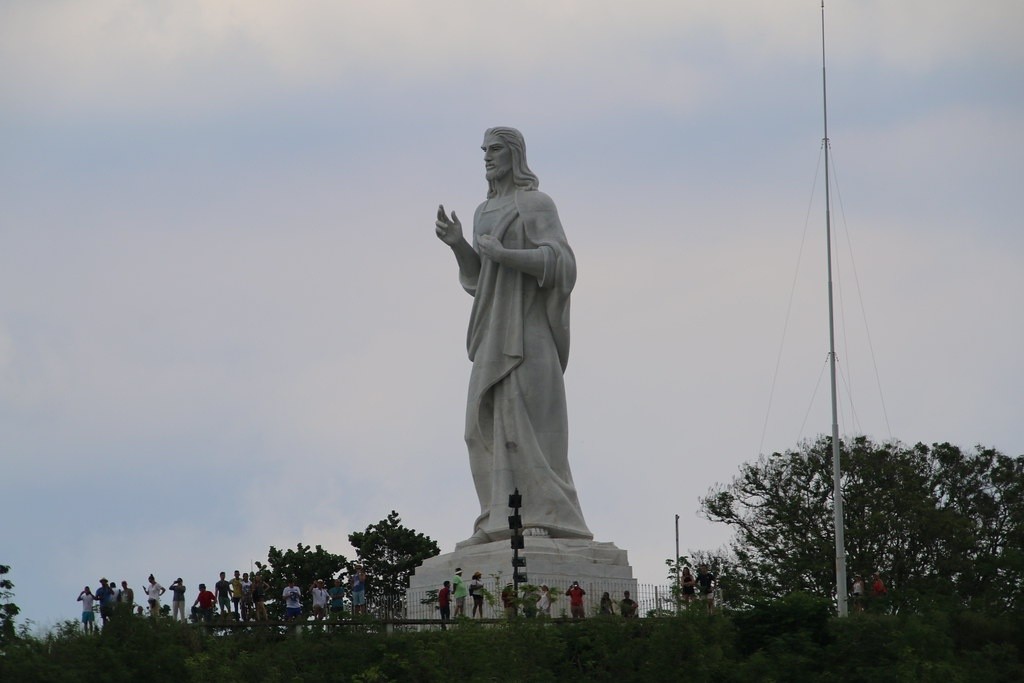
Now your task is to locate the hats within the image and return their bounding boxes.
[539,584,548,588]
[475,572,482,577]
[99,577,108,582]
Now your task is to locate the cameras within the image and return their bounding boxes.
[573,585,577,588]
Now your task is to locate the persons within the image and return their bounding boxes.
[109,581,144,617]
[852,572,888,614]
[438,581,452,630]
[144,573,165,618]
[600,591,616,616]
[681,564,717,615]
[77,586,96,637]
[94,578,114,626]
[435,126,594,551]
[282,563,366,631]
[191,584,217,637]
[451,567,467,619]
[502,582,552,620]
[566,581,586,618]
[169,578,186,624]
[620,591,637,617]
[471,572,484,619]
[215,570,270,622]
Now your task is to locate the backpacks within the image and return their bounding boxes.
[469,579,477,596]
